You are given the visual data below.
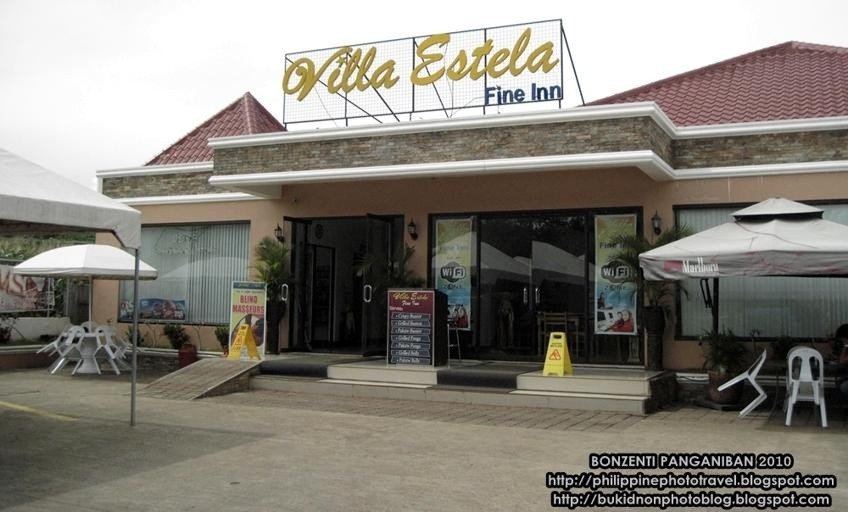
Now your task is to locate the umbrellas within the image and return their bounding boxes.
[636,196,847,332]
[11,241,160,332]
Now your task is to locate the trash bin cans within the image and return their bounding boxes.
[179,343,198,369]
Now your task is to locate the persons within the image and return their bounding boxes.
[452,309,458,320]
[597,292,610,309]
[150,300,185,320]
[611,309,636,332]
[614,311,626,328]
[25,277,43,309]
[448,305,470,328]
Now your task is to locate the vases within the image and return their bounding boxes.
[179,344,197,368]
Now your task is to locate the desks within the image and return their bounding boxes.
[538,315,580,358]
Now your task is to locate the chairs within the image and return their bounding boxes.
[35,321,130,376]
[543,313,595,361]
[716,346,830,427]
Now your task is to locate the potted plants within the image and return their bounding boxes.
[600,222,693,369]
[695,326,754,402]
[214,325,230,355]
[247,236,292,355]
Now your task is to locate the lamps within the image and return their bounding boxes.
[651,210,663,235]
[407,219,417,241]
[274,222,285,243]
